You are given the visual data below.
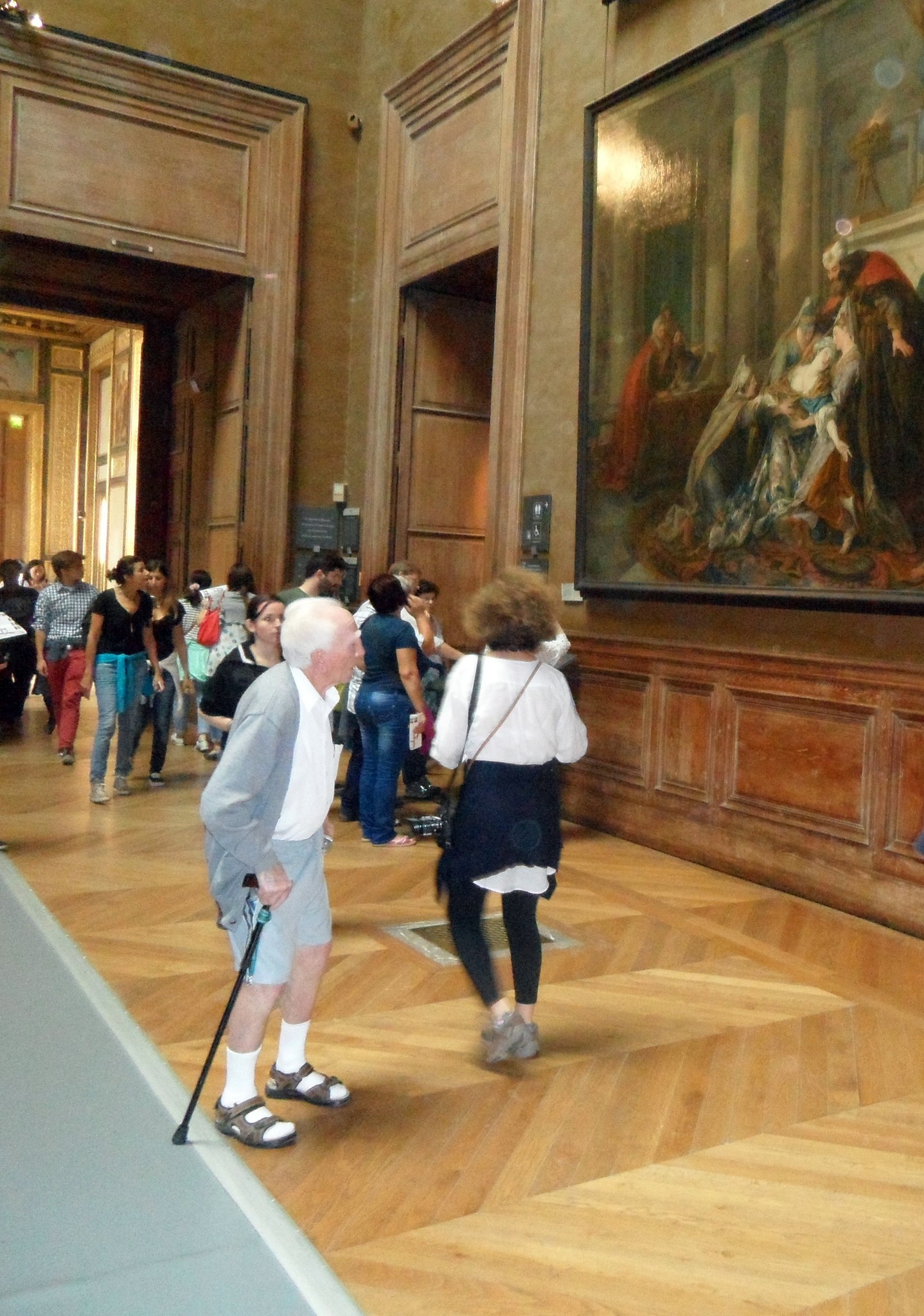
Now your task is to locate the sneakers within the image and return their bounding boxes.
[89,783,108,801]
[111,776,130,794]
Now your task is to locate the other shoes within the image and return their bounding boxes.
[361,838,371,841]
[60,750,75,765]
[171,734,184,746]
[478,1010,540,1061]
[149,772,165,786]
[204,752,217,761]
[373,835,417,847]
[195,741,209,751]
[0,840,10,849]
[44,722,55,734]
[406,776,440,799]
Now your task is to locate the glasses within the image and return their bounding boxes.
[406,572,419,585]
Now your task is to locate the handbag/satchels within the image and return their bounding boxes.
[198,592,224,645]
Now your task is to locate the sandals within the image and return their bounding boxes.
[214,1095,296,1146]
[264,1061,352,1106]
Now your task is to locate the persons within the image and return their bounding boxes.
[0,550,570,847]
[431,565,587,1066]
[201,597,367,1146]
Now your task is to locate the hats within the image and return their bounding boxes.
[51,550,85,565]
